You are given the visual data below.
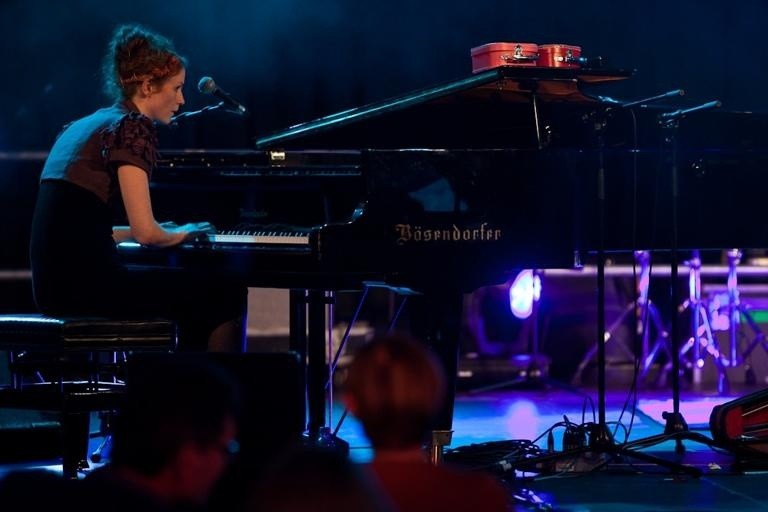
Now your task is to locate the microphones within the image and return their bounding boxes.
[198,77,246,115]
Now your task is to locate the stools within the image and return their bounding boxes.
[0,310,182,477]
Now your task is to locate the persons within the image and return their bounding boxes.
[29,21,245,352]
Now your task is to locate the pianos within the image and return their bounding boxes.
[117,198,461,444]
